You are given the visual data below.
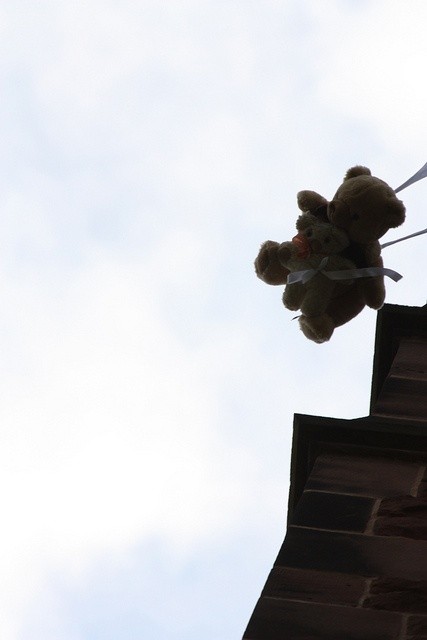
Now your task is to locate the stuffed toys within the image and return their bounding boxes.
[254,166,405,344]
[277,211,357,317]
